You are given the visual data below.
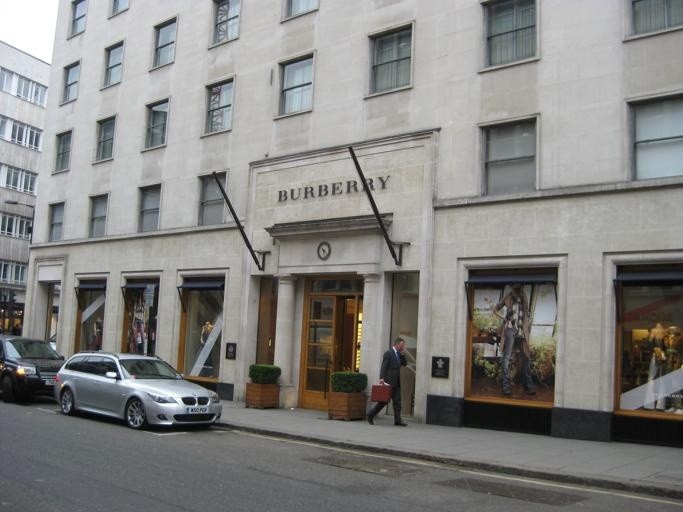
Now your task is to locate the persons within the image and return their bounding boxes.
[491,282,536,395]
[12,323,21,336]
[200,321,215,369]
[130,288,148,355]
[643,321,667,409]
[367,337,409,427]
[666,325,683,417]
[144,307,157,356]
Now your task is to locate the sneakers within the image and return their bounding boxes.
[674,408,682,415]
[664,407,675,413]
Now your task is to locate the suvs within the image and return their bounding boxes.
[0,334,67,402]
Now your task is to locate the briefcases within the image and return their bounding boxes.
[371,381,391,402]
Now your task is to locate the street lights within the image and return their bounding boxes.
[9,291,17,314]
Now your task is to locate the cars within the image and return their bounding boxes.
[54,351,223,430]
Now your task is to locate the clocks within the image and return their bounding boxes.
[317,243,331,261]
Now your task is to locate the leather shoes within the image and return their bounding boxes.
[394,419,406,426]
[526,387,536,394]
[366,411,374,424]
[502,388,512,395]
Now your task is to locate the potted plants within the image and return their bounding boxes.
[326,371,367,423]
[244,362,281,410]
